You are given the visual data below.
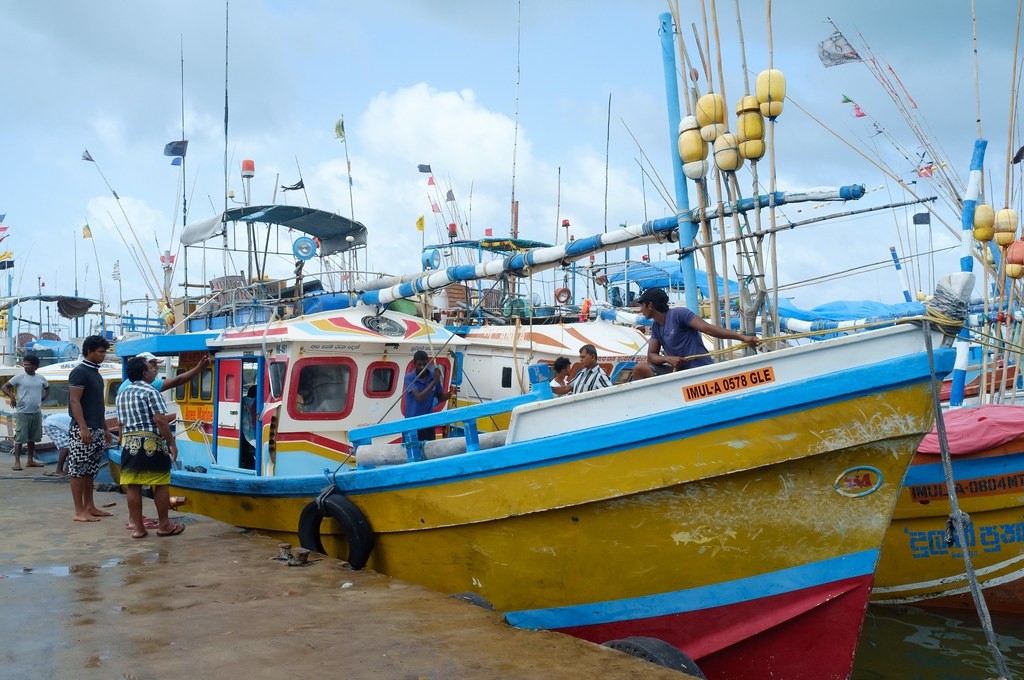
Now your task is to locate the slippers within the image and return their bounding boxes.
[132,528,147,538]
[157,523,185,536]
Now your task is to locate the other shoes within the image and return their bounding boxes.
[126,516,157,529]
[12,466,23,471]
[26,462,43,467]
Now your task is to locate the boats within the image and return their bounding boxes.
[1,4,1024,679]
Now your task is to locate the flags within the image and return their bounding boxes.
[816,32,862,69]
[82,224,92,238]
[335,118,345,138]
[281,179,305,191]
[0,214,14,271]
[164,141,188,166]
[486,229,492,236]
[81,150,94,161]
[415,164,454,230]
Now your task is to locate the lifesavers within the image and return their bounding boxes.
[296,492,375,570]
[141,485,154,499]
[557,288,571,303]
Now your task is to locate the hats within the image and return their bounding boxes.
[637,287,669,312]
[136,352,164,363]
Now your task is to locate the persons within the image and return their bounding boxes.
[66,335,114,522]
[551,344,613,395]
[43,413,72,476]
[0,354,50,471]
[632,288,762,380]
[117,351,211,530]
[549,357,571,398]
[402,350,454,443]
[115,356,185,539]
